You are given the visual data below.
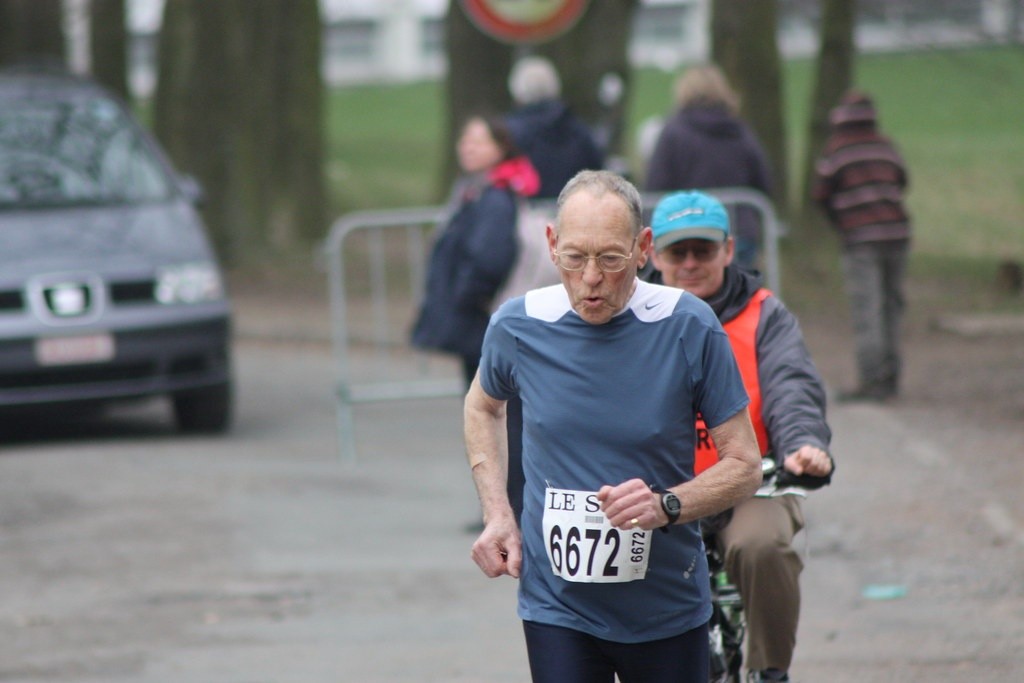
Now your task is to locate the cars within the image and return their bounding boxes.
[0,71,231,430]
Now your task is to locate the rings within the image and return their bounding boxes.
[631,517,639,529]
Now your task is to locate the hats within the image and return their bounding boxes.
[650,189,730,253]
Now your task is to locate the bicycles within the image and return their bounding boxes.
[696,454,808,683]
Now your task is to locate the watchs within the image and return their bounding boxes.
[648,484,682,529]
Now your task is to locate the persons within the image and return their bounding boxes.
[637,65,780,286]
[404,109,563,534]
[490,53,609,201]
[630,189,837,683]
[804,95,913,404]
[462,166,763,682]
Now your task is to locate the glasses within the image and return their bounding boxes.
[552,236,639,273]
[662,243,722,264]
